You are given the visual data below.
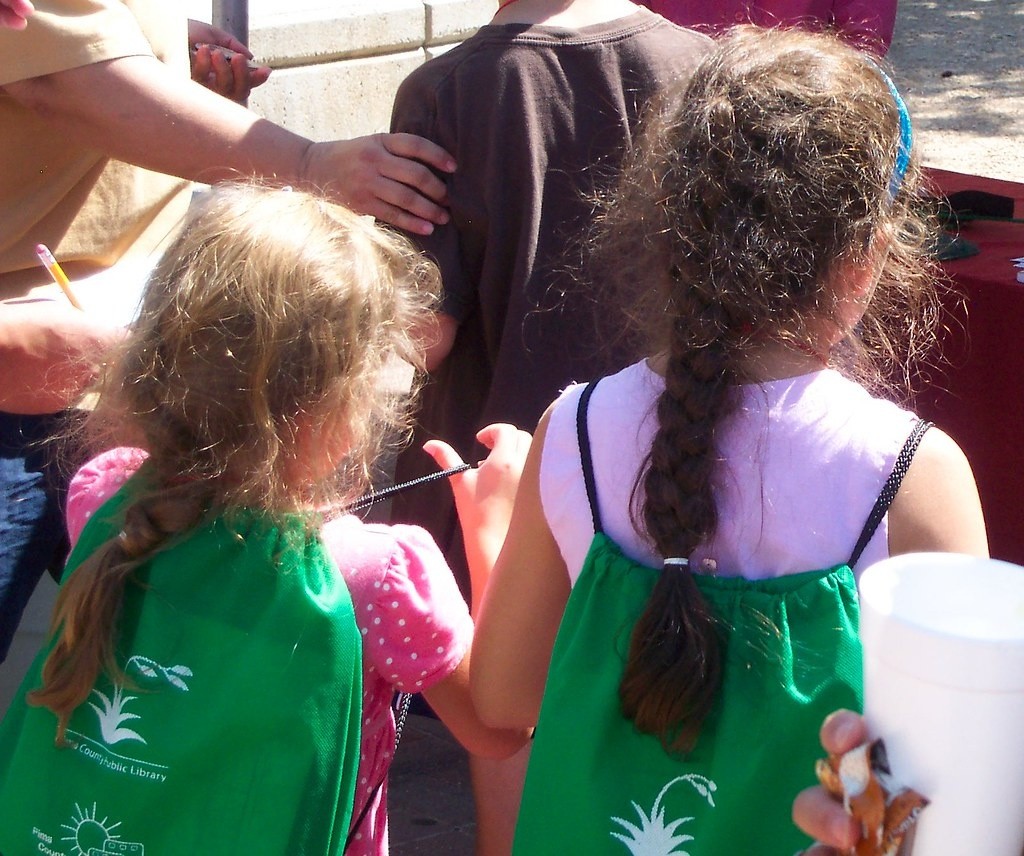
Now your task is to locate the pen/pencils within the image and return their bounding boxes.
[35,243,84,310]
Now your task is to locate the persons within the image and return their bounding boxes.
[793,708,871,856]
[468,26,991,856]
[0,0,899,856]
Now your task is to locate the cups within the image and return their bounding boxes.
[854,550,1024,856]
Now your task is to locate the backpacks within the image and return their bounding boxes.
[511,381,934,856]
[0,463,471,856]
